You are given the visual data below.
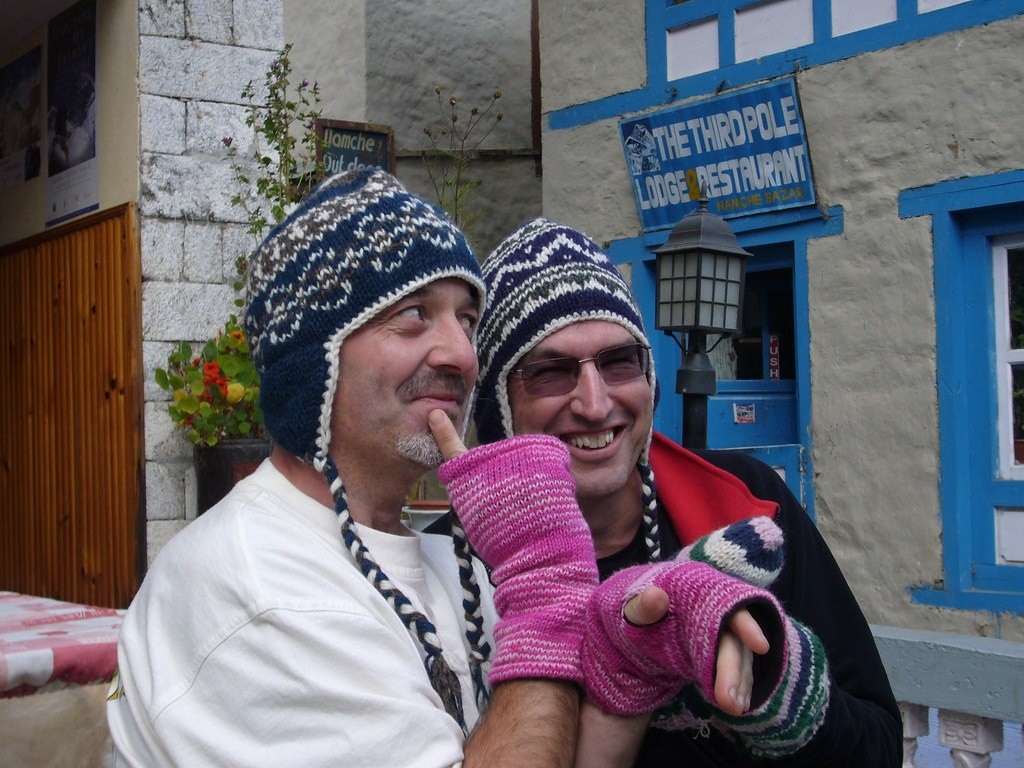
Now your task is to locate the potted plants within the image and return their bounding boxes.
[193,439,273,517]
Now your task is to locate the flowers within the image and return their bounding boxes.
[156,315,263,447]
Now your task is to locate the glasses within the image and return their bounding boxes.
[510,343,652,399]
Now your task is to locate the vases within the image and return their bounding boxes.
[401,500,451,531]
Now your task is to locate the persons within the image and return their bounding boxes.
[105,165,792,768]
[477,215,906,768]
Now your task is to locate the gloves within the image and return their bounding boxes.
[650,516,831,760]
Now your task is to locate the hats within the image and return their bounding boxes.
[244,166,492,736]
[474,219,662,563]
